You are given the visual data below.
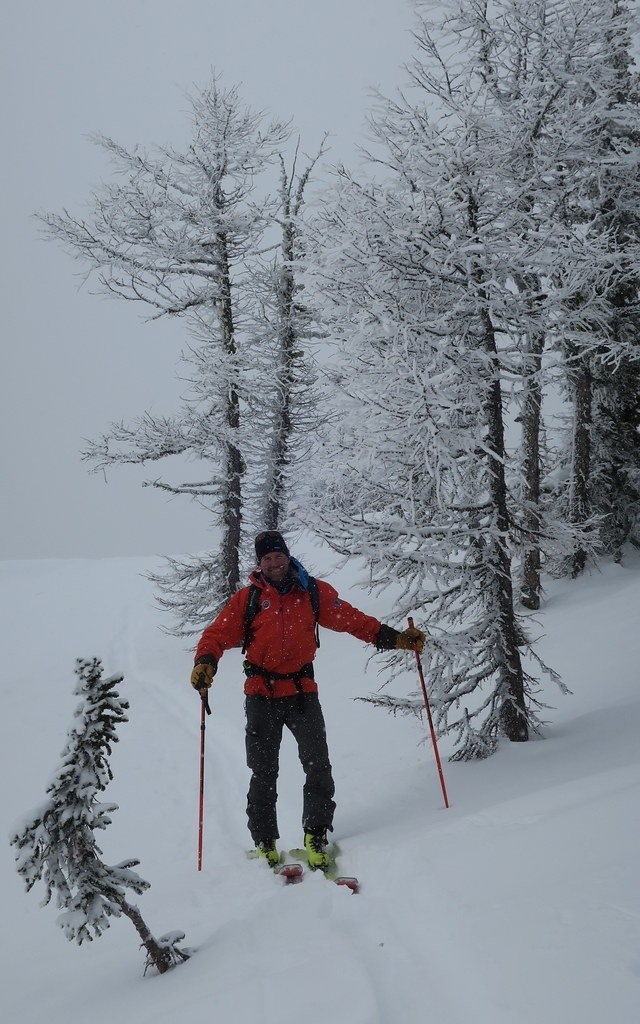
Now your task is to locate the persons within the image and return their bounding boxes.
[192,531,425,872]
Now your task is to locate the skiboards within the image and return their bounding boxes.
[249,845,361,889]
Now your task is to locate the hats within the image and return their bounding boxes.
[255,531,290,564]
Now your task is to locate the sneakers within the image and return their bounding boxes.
[257,837,278,867]
[303,830,331,870]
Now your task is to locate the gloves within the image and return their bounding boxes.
[396,627,426,652]
[190,663,214,690]
